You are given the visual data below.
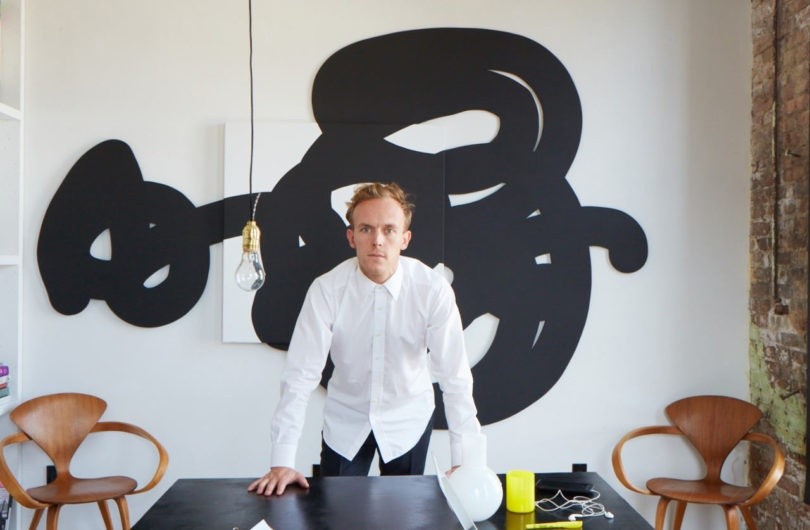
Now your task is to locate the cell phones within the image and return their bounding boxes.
[535,479,594,492]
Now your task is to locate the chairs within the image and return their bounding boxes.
[0,393,169,530]
[612,395,785,530]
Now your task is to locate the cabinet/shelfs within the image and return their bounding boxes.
[0,0,22,530]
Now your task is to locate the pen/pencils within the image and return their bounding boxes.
[525,521,582,529]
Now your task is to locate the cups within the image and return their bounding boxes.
[506,470,536,513]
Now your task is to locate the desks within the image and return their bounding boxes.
[130,472,655,530]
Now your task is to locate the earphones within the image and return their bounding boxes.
[604,511,614,519]
[568,514,582,521]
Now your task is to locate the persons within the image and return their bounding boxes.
[248,184,485,496]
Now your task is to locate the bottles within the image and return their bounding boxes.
[443,432,504,523]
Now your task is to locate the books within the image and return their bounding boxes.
[0,364,10,398]
